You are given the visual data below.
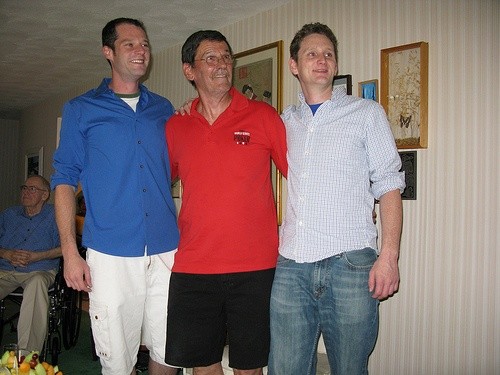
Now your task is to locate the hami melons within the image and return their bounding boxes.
[6,351,63,375]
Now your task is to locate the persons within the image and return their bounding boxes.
[49,18,180,375]
[0,175,62,363]
[165,30,378,375]
[174,22,406,375]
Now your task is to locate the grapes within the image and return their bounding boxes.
[29,360,38,369]
[32,353,38,360]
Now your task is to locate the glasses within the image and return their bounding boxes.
[186,55,236,66]
[21,185,48,193]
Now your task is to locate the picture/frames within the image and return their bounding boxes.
[25,146,43,181]
[231,40,282,226]
[381,40,430,149]
[375,151,417,202]
[357,79,379,104]
[333,75,352,95]
